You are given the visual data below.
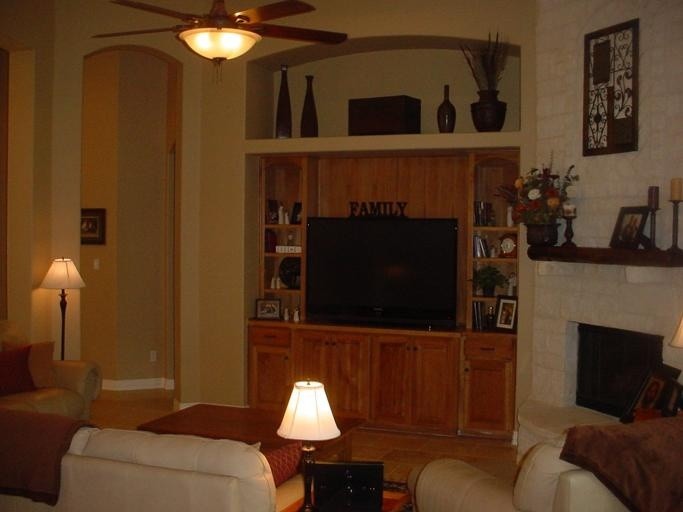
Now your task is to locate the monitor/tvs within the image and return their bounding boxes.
[305,218,458,332]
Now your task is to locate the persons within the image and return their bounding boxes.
[630,217,640,240]
[641,381,660,407]
[622,215,635,240]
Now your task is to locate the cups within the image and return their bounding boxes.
[474,201,493,226]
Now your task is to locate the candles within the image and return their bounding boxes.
[647,185,660,212]
[670,177,683,202]
[561,201,577,217]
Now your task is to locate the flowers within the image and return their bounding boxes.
[491,149,582,224]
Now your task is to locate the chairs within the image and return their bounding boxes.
[412,416,683,512]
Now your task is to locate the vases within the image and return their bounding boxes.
[275,63,319,137]
[524,224,558,247]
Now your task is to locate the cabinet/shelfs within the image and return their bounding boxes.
[247,323,517,441]
[464,149,518,331]
[257,153,307,322]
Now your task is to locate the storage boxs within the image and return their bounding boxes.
[347,93,421,137]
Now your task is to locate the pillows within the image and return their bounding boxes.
[0,339,57,397]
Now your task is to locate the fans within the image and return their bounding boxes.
[90,0,348,62]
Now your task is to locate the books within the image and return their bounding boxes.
[471,234,489,331]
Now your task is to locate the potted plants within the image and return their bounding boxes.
[472,264,510,297]
[457,30,513,133]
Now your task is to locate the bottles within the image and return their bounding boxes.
[300,75,318,137]
[293,308,300,322]
[267,199,302,225]
[275,62,292,138]
[270,276,281,289]
[485,306,498,328]
[283,308,290,320]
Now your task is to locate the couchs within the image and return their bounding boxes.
[0,319,103,420]
[0,405,275,512]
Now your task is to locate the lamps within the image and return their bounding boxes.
[273,378,342,512]
[37,257,87,361]
[175,0,262,65]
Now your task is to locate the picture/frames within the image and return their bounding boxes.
[618,358,682,425]
[491,295,519,333]
[608,204,649,249]
[79,207,107,245]
[256,298,282,321]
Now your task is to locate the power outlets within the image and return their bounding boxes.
[149,350,157,362]
